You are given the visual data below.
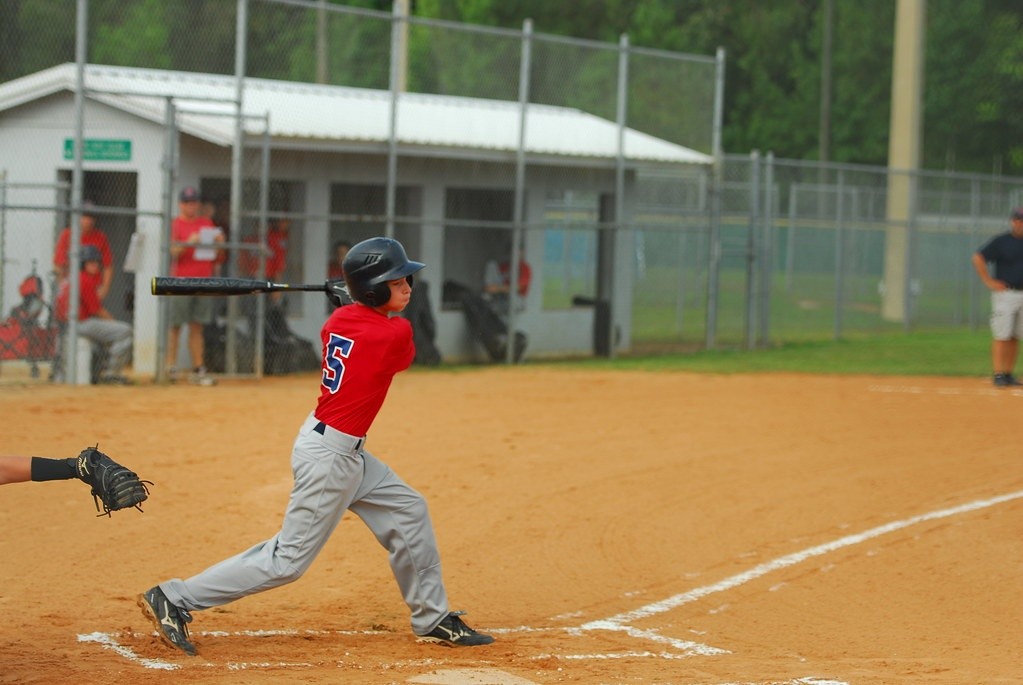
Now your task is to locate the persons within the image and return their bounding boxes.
[328,240,352,312]
[0,442,154,518]
[441,281,527,364]
[485,241,530,316]
[135,237,497,656]
[972,211,1023,387]
[0,201,132,385]
[164,187,288,387]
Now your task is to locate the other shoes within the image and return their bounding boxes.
[165,370,181,383]
[104,369,135,385]
[190,370,217,386]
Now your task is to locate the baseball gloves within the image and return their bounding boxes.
[74,443,156,519]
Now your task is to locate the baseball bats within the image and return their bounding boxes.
[151,275,328,297]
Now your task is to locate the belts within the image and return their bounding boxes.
[314,422,361,452]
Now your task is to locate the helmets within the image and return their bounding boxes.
[343,237,426,308]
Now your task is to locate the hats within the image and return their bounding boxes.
[179,187,201,203]
[82,245,101,262]
[82,201,98,218]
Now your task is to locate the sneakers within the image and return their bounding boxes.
[415,611,495,648]
[994,374,1007,387]
[1006,373,1023,386]
[138,586,197,658]
